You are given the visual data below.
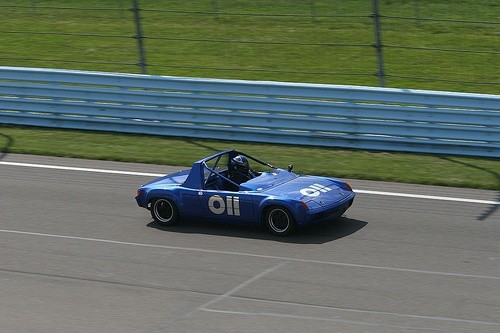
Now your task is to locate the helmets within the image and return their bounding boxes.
[230,155,249,177]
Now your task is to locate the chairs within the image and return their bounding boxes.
[215,170,239,192]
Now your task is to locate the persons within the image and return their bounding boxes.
[231,156,260,190]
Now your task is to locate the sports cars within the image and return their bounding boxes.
[136,150,355,234]
